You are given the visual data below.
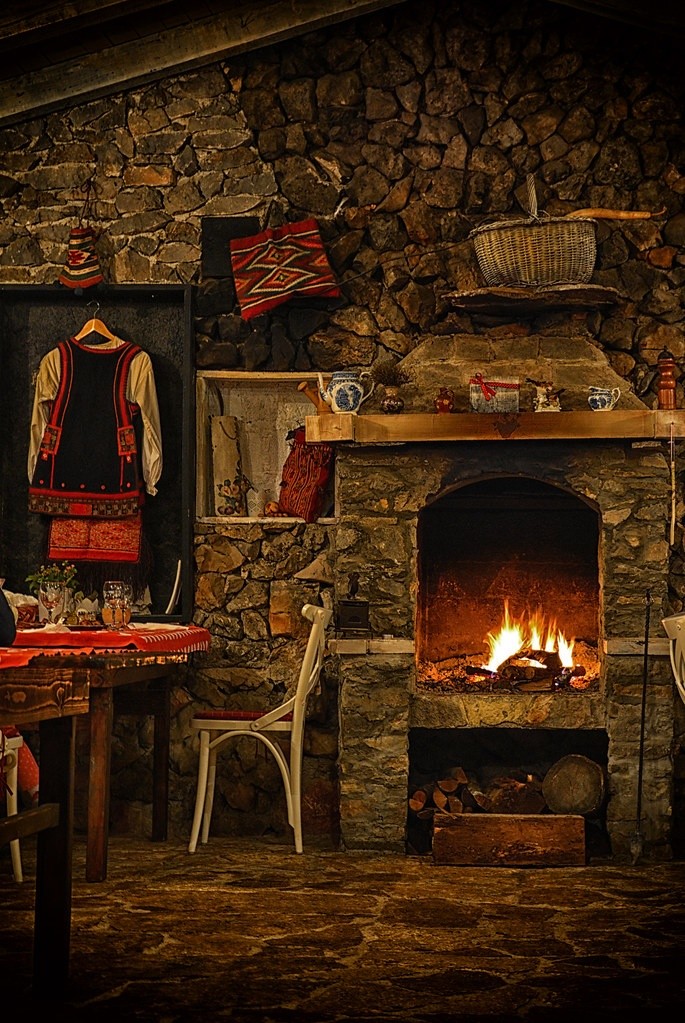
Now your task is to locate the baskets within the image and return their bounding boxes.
[466,172,598,287]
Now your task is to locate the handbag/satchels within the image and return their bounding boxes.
[279,423,336,524]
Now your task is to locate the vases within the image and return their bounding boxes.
[38,582,65,625]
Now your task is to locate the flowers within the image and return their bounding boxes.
[24,558,78,596]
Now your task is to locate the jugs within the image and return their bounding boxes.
[317,371,375,416]
[588,385,620,412]
[298,380,334,416]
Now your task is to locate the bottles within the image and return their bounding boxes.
[657,344,677,410]
[433,387,455,414]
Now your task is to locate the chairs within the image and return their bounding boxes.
[188,603,334,856]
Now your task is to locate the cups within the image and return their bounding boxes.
[102,581,126,633]
[124,585,132,624]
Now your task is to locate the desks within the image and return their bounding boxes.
[0,643,185,1023]
[0,622,209,882]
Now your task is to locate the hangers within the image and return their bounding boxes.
[76,299,115,341]
